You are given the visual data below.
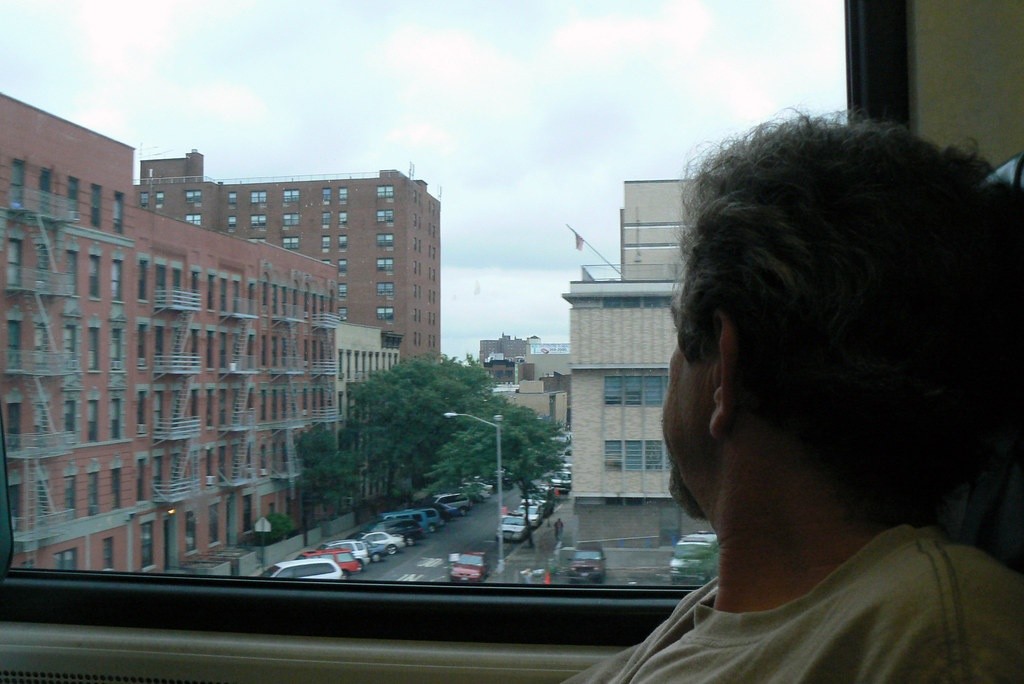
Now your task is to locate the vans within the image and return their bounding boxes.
[565,541,607,584]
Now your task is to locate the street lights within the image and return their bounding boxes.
[444,412,505,574]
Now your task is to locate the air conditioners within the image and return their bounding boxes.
[137,424,148,434]
[261,469,268,475]
[206,476,216,485]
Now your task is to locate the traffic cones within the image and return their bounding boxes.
[544,571,551,584]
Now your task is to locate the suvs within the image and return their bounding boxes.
[668,530,718,586]
[317,539,371,567]
[257,557,348,580]
[294,548,363,577]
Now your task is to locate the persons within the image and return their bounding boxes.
[554,517,563,539]
[535,106,1024,684]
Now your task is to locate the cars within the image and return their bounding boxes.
[450,551,491,583]
[495,430,573,543]
[343,473,493,564]
